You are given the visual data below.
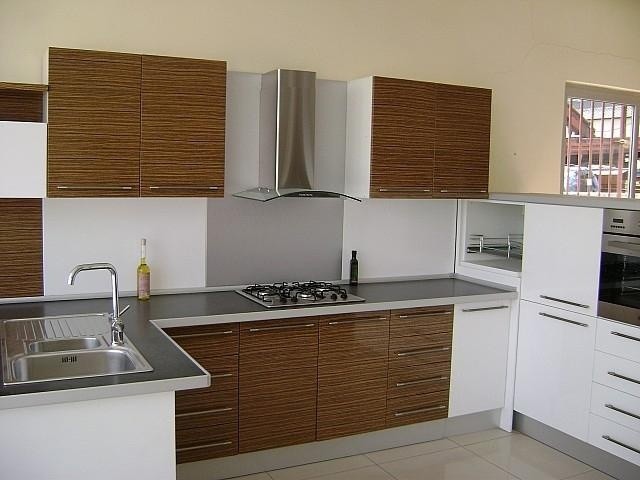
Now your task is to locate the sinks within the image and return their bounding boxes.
[2,347,154,385]
[26,334,107,355]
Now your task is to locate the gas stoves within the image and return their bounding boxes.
[237,280,365,309]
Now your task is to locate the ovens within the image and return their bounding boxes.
[597,208,639,325]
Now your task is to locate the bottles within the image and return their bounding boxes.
[350,248,360,287]
[137,236,151,302]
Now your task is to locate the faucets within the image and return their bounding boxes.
[67,263,132,347]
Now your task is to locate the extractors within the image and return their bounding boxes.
[233,70,362,205]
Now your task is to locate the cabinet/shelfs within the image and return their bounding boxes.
[391,306,454,427]
[47,47,226,200]
[346,78,493,200]
[242,315,388,452]
[518,203,604,318]
[164,324,240,464]
[448,298,510,420]
[463,199,526,274]
[513,299,596,444]
[587,317,640,467]
